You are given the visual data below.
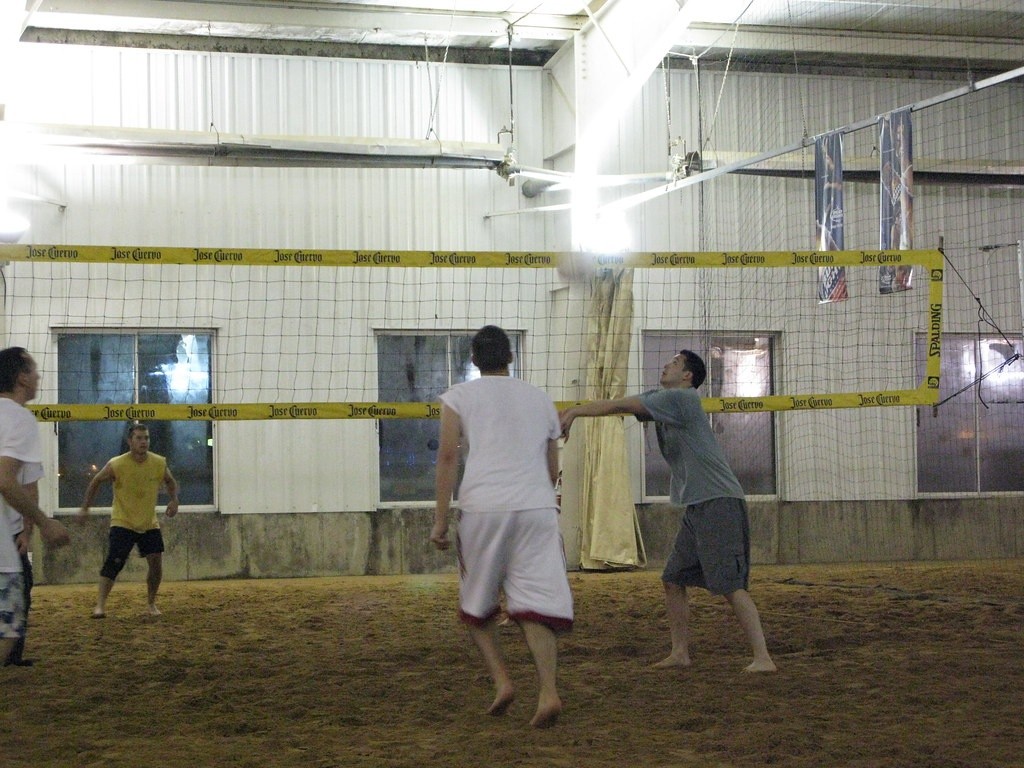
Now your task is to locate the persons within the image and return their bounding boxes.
[558,349,778,673]
[429,325,575,730]
[76,422,182,619]
[0,344,72,668]
